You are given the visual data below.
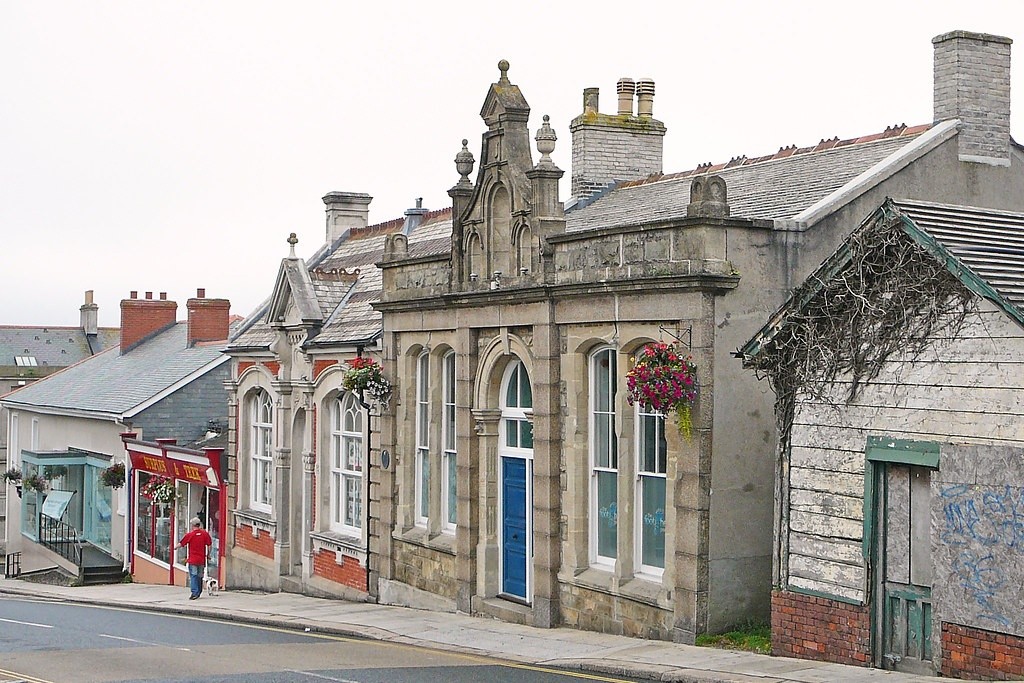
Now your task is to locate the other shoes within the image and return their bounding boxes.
[189,593,200,600]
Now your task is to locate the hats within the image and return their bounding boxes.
[190,517,200,525]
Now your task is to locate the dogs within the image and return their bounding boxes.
[205,577,218,596]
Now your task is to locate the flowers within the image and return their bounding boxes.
[621,343,700,444]
[100,462,125,489]
[141,476,175,504]
[341,357,394,413]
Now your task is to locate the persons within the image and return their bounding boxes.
[174,517,213,600]
